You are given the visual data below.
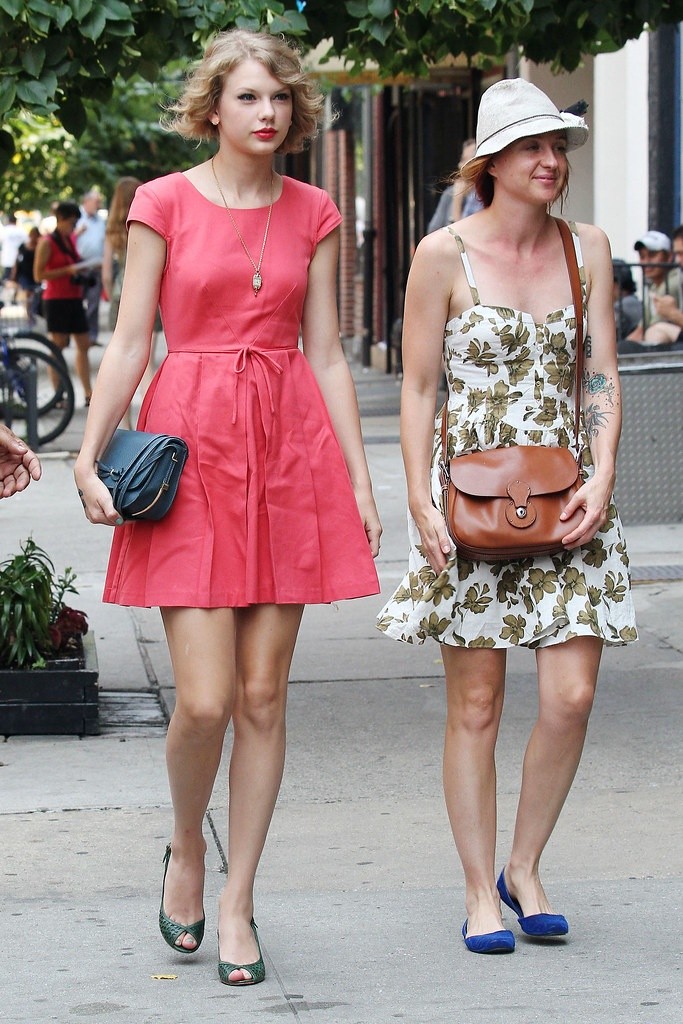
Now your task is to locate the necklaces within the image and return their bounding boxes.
[211,154,274,296]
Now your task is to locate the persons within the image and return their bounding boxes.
[0,214,43,325]
[102,176,164,431]
[612,225,683,357]
[0,422,42,499]
[424,138,487,238]
[34,203,94,407]
[74,184,108,347]
[74,32,382,986]
[377,78,640,956]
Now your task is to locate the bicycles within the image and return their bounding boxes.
[0,307,76,452]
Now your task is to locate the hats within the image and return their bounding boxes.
[634,230,671,252]
[460,78,590,181]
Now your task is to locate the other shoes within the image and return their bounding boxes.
[462,919,515,953]
[497,865,568,937]
[54,399,66,408]
[86,396,91,405]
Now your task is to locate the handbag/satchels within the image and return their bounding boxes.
[441,446,587,560]
[96,428,187,520]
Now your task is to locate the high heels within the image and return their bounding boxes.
[158,842,205,953]
[219,917,265,985]
